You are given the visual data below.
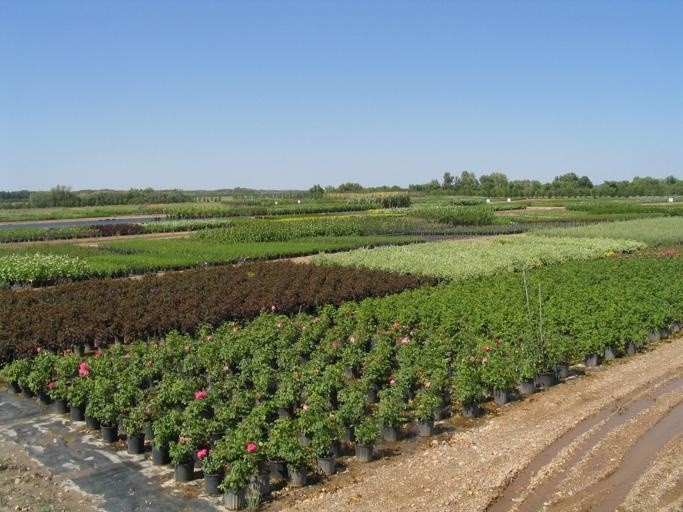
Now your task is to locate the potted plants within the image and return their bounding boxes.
[0,259,683,509]
